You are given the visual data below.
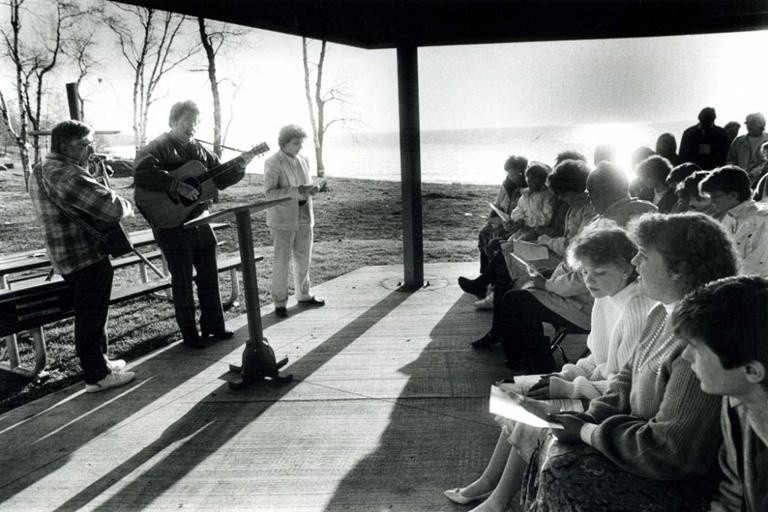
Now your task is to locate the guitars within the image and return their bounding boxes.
[88,153,134,257]
[133,141,271,232]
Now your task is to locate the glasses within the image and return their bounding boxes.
[65,141,97,153]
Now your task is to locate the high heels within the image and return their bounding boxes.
[444,485,495,505]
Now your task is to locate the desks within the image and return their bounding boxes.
[1,215,231,271]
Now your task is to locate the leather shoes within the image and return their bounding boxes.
[202,331,234,338]
[275,306,286,315]
[459,277,487,298]
[504,359,543,371]
[184,335,203,349]
[472,340,498,353]
[298,295,324,306]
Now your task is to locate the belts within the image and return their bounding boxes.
[299,200,307,207]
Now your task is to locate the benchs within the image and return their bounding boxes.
[1,251,272,376]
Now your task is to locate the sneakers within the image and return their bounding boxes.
[474,292,494,309]
[103,354,126,370]
[85,370,135,393]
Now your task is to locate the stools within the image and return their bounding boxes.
[544,318,593,364]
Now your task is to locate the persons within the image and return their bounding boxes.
[498,162,659,375]
[134,100,254,346]
[444,226,669,510]
[478,154,528,274]
[263,124,325,317]
[635,107,768,275]
[554,152,588,169]
[28,120,135,393]
[664,275,768,512]
[457,160,551,309]
[469,159,592,354]
[520,212,742,510]
